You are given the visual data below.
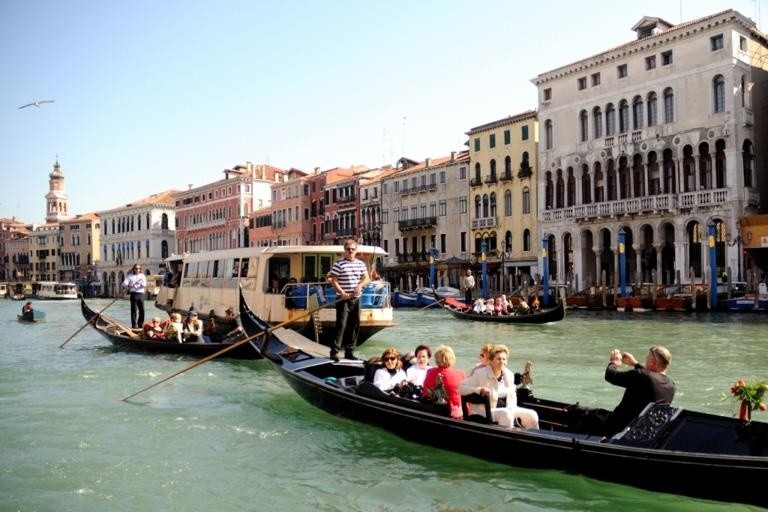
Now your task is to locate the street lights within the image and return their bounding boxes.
[494,240,512,296]
[725,220,752,291]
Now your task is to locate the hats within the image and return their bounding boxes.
[188,311,197,316]
[152,317,160,322]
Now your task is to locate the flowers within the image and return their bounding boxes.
[717,377,768,427]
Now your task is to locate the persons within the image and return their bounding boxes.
[22,301,34,315]
[462,269,476,307]
[360,340,543,431]
[370,275,385,305]
[309,275,328,304]
[285,277,301,309]
[120,263,148,329]
[171,269,182,286]
[158,267,173,287]
[265,277,285,294]
[563,343,677,438]
[329,238,371,362]
[470,294,541,316]
[142,308,242,344]
[288,278,322,309]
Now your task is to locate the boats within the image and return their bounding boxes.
[77,289,265,360]
[564,281,768,314]
[153,245,395,348]
[233,283,766,511]
[31,281,79,300]
[23,308,46,323]
[393,285,476,308]
[430,283,565,324]
[0,281,33,301]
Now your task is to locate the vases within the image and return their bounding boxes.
[740,402,749,421]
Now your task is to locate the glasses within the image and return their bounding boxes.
[649,346,655,357]
[382,356,395,361]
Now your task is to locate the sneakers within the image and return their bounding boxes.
[330,357,339,362]
[345,356,358,360]
